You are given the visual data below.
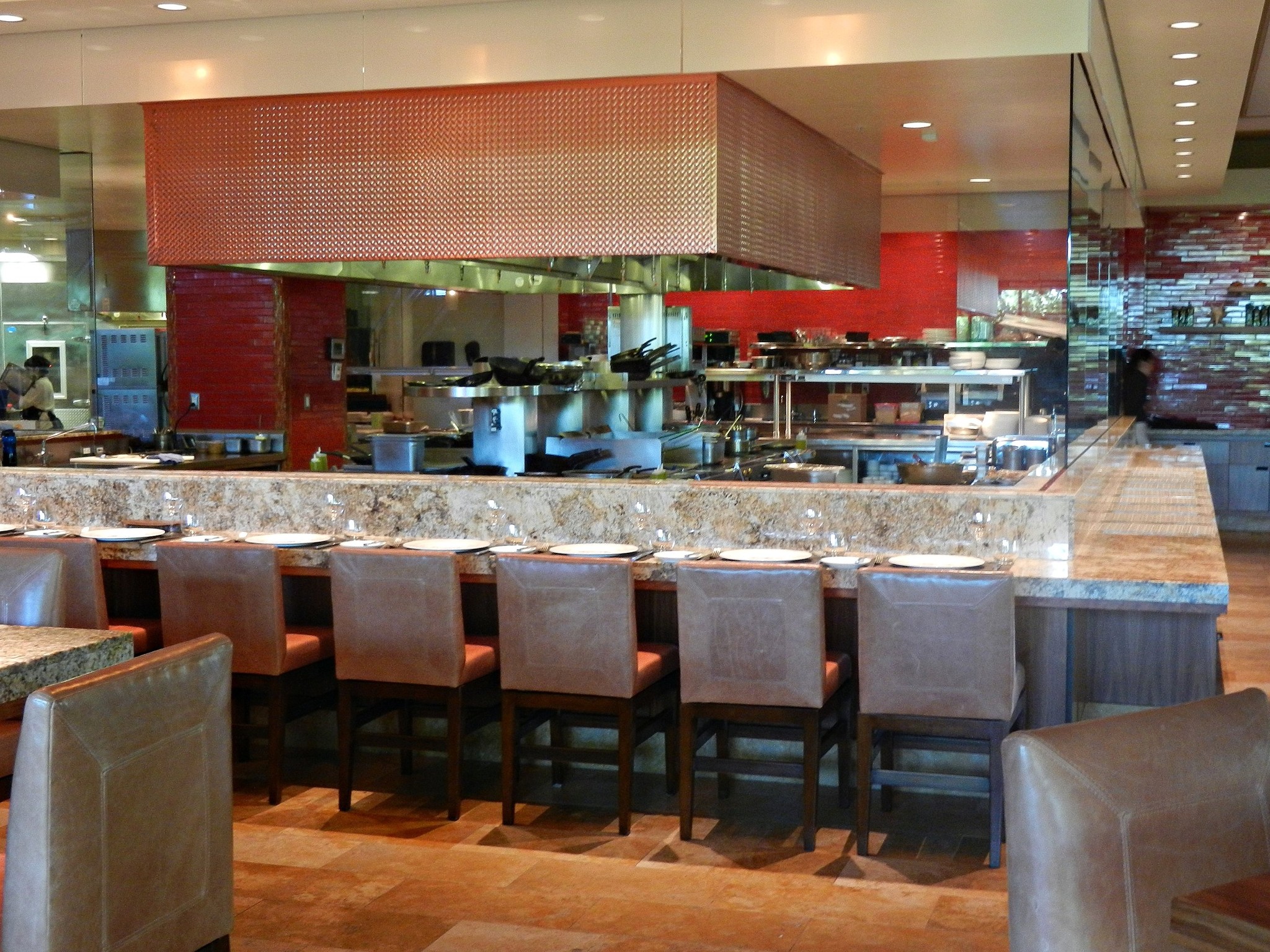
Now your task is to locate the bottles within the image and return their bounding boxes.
[1170,304,1194,328]
[796,430,807,450]
[2,428,17,467]
[310,446,330,474]
[1245,303,1270,326]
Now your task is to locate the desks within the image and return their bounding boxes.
[69,450,286,471]
[0,624,134,803]
[1170,874,1270,952]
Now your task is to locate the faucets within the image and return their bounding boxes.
[35,422,98,467]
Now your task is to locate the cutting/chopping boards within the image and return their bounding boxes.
[71,455,196,462]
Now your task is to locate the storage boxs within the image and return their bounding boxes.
[359,433,433,473]
[546,430,703,475]
[829,394,869,421]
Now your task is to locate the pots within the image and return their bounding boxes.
[665,423,758,464]
[515,448,657,477]
[447,336,701,384]
[453,456,508,476]
[320,449,372,465]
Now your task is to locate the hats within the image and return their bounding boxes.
[24,355,51,367]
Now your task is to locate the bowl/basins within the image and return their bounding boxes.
[719,351,834,369]
[946,426,978,440]
[863,460,963,486]
[195,441,226,454]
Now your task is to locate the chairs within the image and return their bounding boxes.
[1000,688,1270,952]
[0,631,235,952]
[0,537,1027,870]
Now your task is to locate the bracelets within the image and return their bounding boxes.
[5,388,9,391]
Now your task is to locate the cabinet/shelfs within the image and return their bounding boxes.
[1159,287,1270,333]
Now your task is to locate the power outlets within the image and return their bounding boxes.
[189,393,200,411]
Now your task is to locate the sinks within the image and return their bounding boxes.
[31,463,133,470]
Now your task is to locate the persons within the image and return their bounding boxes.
[1119,348,1156,444]
[0,355,56,421]
[1017,337,1066,415]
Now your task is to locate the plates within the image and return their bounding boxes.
[0,524,986,569]
[980,410,1048,438]
[882,328,1022,369]
